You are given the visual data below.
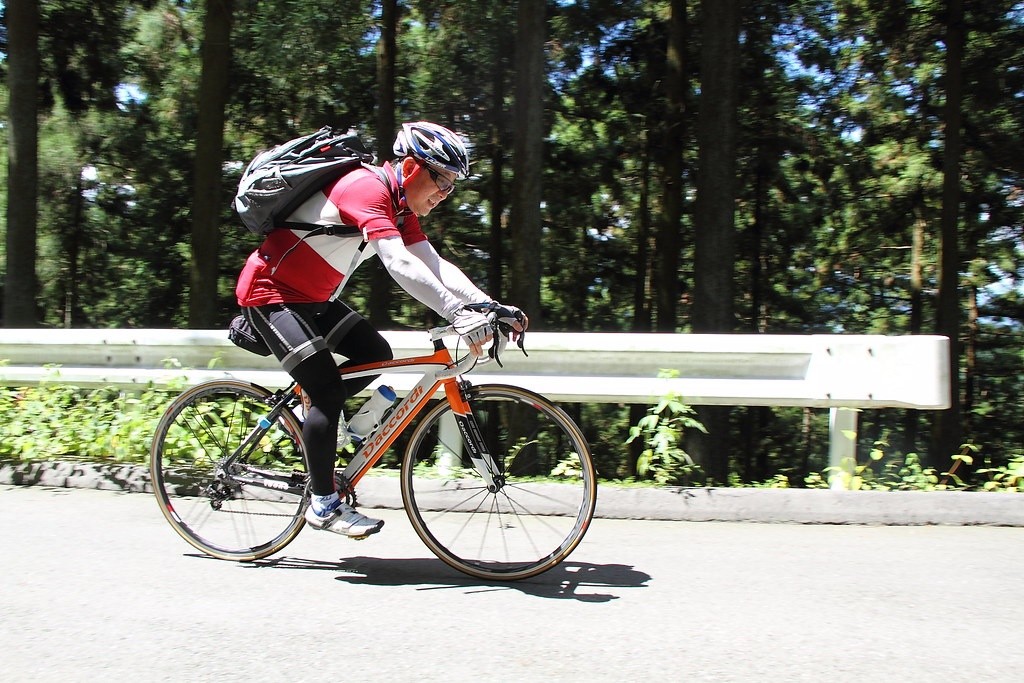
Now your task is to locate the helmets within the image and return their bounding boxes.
[393,122,470,181]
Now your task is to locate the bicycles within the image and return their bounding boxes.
[149,301,598,581]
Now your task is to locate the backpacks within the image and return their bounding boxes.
[231,124,406,235]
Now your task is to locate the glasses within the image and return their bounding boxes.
[412,156,457,195]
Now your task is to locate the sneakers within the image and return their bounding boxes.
[300,387,351,448]
[304,502,385,538]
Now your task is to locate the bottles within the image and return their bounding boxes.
[336,407,351,451]
[347,385,397,441]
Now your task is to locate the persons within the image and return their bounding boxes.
[236,122,529,536]
